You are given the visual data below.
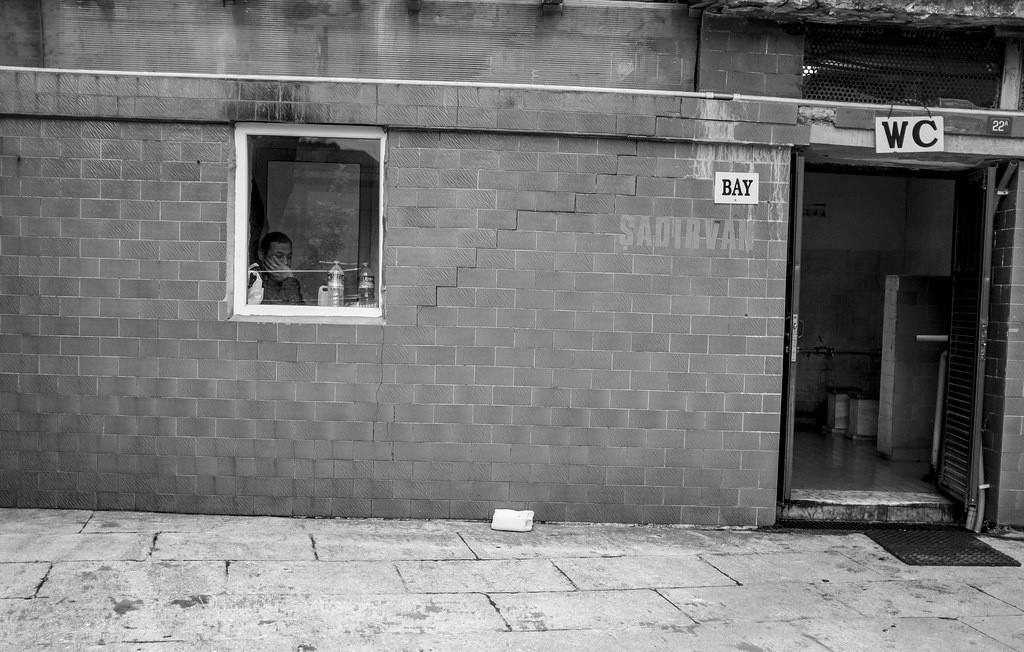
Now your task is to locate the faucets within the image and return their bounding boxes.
[827,345,835,358]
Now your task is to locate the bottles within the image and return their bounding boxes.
[357,262,379,305]
[317,285,328,306]
[327,261,344,307]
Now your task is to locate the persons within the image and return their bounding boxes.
[250,232,308,304]
